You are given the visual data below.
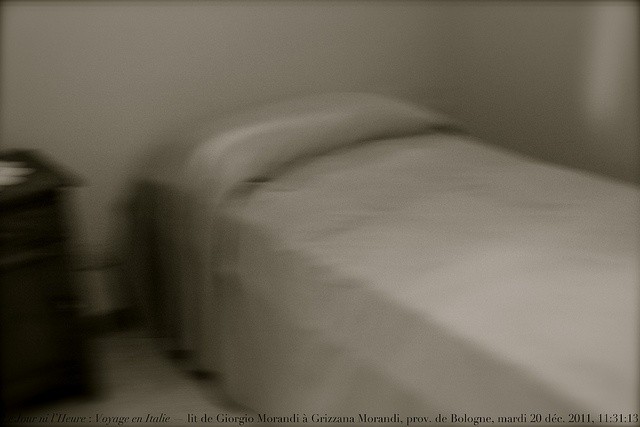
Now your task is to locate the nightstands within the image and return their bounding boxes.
[1,149,105,419]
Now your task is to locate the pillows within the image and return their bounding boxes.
[143,90,465,205]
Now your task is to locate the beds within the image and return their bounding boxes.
[134,131,640,427]
